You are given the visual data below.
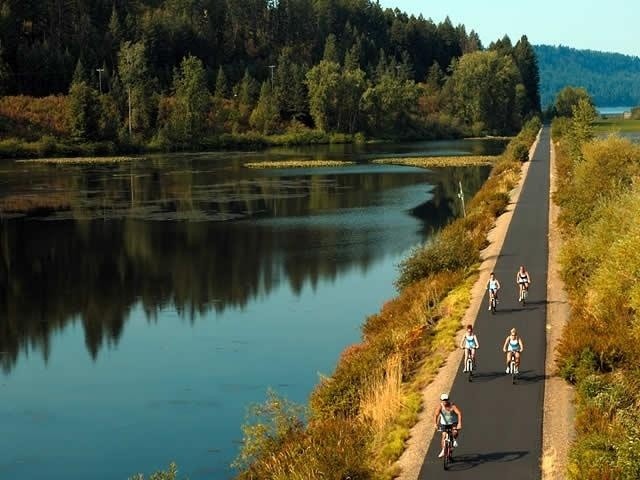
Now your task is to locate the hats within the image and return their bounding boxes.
[440,394,449,401]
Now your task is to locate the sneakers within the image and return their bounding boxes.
[506,366,509,374]
[453,441,458,447]
[438,451,444,457]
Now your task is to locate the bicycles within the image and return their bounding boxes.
[463,347,477,382]
[437,428,457,469]
[505,350,520,386]
[519,281,528,307]
[488,287,497,313]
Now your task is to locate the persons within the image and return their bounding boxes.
[485,273,500,311]
[502,328,524,374]
[433,394,462,458]
[460,325,480,373]
[517,266,531,302]
